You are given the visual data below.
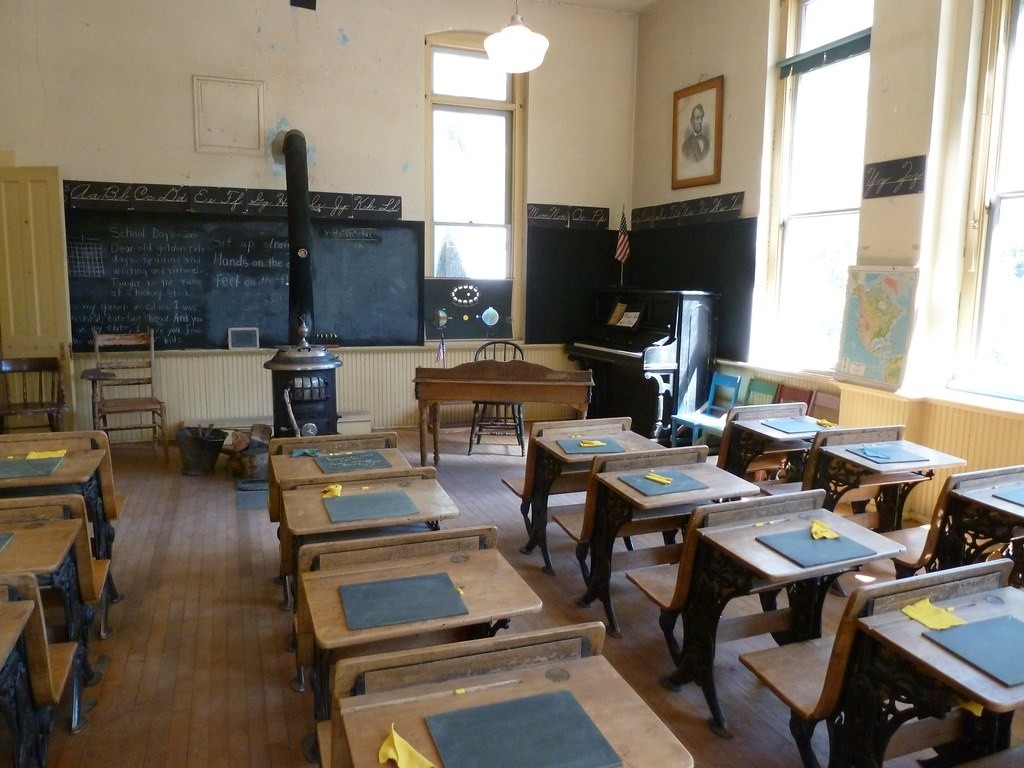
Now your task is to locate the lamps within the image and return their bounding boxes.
[483,0,550,73]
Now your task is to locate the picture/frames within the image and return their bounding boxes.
[227,327,260,349]
[671,75,726,189]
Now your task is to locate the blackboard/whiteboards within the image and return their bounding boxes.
[623,217,758,362]
[65,205,425,354]
[524,217,621,344]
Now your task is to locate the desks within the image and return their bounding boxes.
[941,473,1024,566]
[79,368,118,381]
[0,519,100,735]
[576,462,759,638]
[272,447,413,610]
[300,550,547,765]
[662,508,906,741]
[817,438,968,533]
[0,448,124,637]
[281,478,459,657]
[722,416,837,501]
[518,429,668,577]
[1,600,34,672]
[826,584,1024,768]
[329,654,695,768]
[412,357,595,479]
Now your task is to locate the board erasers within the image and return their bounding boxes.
[326,344,339,348]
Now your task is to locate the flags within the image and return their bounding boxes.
[437,342,443,361]
[615,212,630,264]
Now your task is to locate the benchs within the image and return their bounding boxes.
[0,394,1024,768]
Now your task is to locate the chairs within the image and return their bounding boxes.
[467,342,527,453]
[771,386,817,415]
[83,330,169,456]
[702,376,779,455]
[671,372,742,452]
[0,356,66,434]
[805,390,839,424]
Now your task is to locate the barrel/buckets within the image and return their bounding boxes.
[174,427,229,476]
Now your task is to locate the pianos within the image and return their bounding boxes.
[564,285,720,443]
